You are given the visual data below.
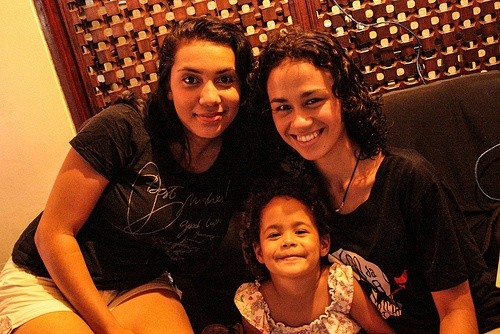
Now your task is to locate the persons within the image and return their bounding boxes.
[247,25,500,334]
[233,180,396,334]
[0,14,258,334]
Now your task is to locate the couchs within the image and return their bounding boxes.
[204,70,500,308]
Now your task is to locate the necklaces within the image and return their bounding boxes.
[334,149,362,212]
